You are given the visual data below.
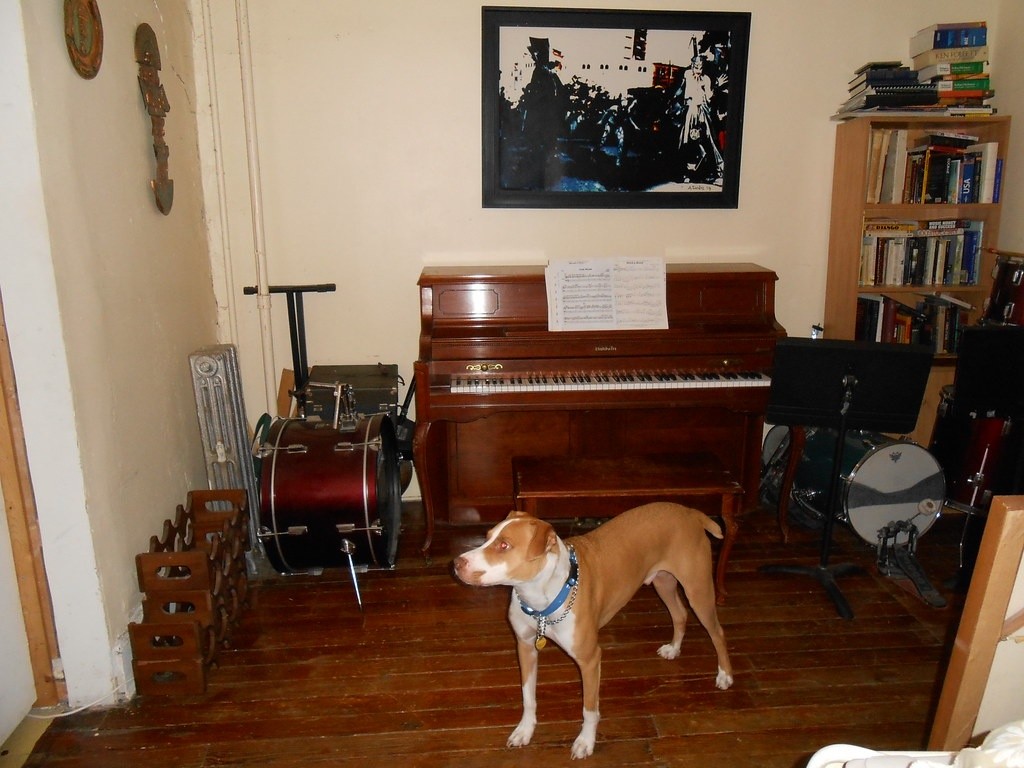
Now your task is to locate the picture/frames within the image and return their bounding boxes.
[480,4,753,211]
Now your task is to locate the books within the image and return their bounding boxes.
[909,20,998,118]
[858,216,985,287]
[829,59,951,122]
[865,127,1003,205]
[856,292,977,354]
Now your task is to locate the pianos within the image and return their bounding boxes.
[410,260,790,561]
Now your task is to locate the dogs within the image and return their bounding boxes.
[454,501,735,761]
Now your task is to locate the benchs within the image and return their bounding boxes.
[505,454,749,610]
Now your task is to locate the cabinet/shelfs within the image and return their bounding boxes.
[821,114,1017,448]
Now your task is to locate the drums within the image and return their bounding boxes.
[247,383,409,610]
[761,420,952,578]
